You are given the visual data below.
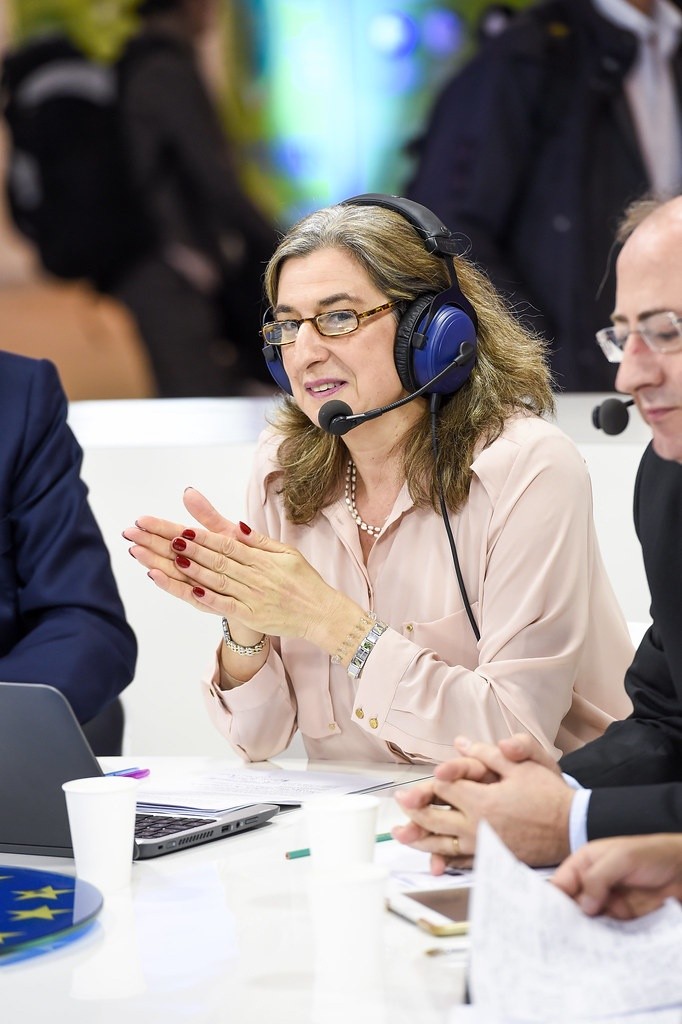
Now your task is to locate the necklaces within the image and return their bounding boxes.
[344,457,380,538]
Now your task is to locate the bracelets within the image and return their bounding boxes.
[330,610,377,664]
[348,621,388,679]
[222,617,269,657]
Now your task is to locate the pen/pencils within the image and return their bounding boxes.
[425,948,466,957]
[285,832,392,861]
[104,766,150,780]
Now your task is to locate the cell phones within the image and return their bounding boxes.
[385,884,472,934]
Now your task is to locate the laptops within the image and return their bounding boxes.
[0,681,281,860]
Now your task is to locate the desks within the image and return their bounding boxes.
[0,760,682,1024]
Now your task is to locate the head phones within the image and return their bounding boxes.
[260,192,479,403]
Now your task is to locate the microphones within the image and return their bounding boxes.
[318,362,457,435]
[592,398,635,436]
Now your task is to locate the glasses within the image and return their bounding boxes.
[261,297,405,346]
[595,311,682,364]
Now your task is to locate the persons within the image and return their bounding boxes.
[409,1,682,395]
[389,189,682,875]
[0,0,287,398]
[0,348,138,755]
[123,195,637,772]
[548,832,682,920]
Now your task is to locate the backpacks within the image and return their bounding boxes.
[0,30,215,295]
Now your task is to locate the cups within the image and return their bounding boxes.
[301,792,380,888]
[61,777,140,893]
